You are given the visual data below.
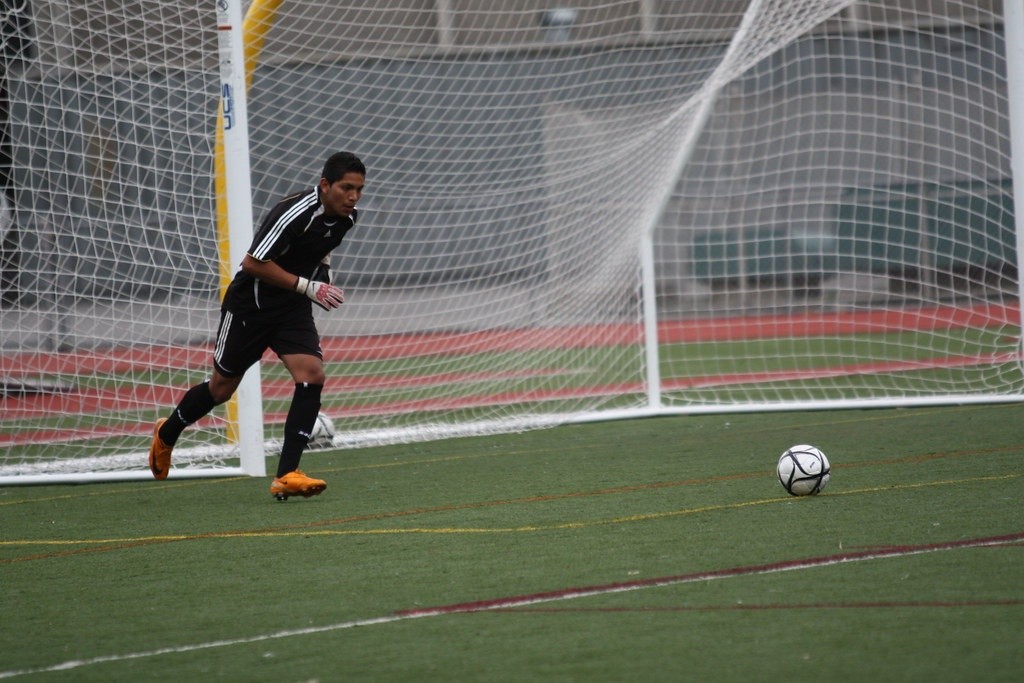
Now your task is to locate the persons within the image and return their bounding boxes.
[147,151,364,502]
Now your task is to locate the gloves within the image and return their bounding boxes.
[294,276,344,311]
[314,262,332,285]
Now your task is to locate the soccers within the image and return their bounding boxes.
[303,411,335,446]
[775,443,831,497]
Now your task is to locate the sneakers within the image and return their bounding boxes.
[270,468,327,501]
[149,417,173,480]
